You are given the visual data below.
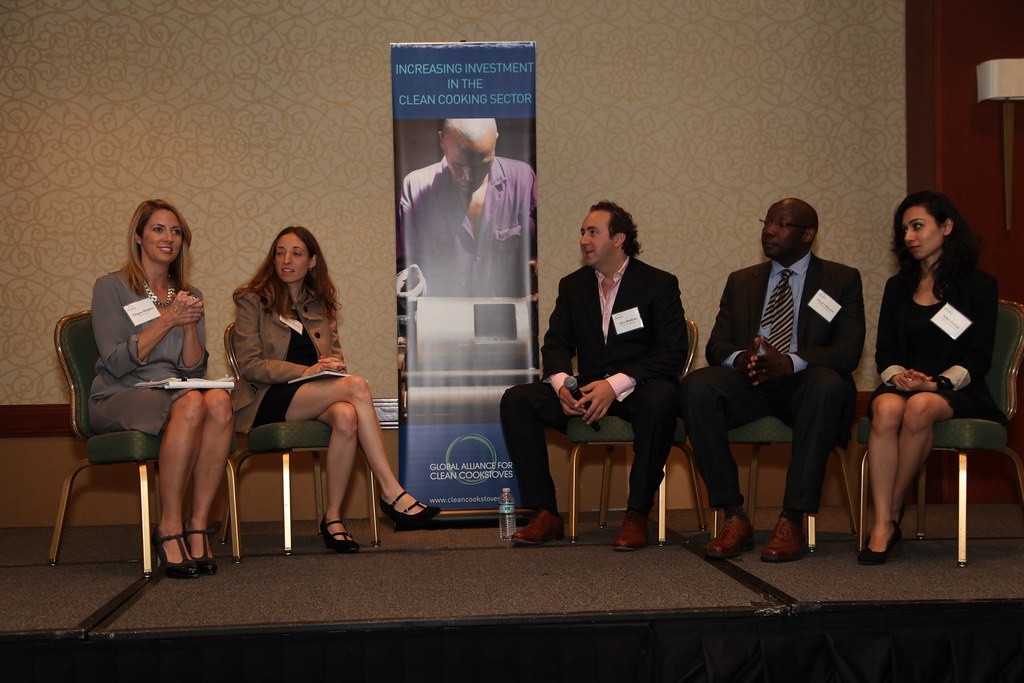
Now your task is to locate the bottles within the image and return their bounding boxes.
[498,488,516,538]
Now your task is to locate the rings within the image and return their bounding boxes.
[763,369,766,374]
[190,296,196,299]
[896,386,900,389]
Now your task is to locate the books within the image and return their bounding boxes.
[288,370,367,386]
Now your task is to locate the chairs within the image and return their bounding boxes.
[566,318,706,548]
[710,411,859,555]
[219,322,381,557]
[856,298,1024,568]
[46,311,242,578]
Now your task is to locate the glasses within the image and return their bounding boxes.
[759,218,808,230]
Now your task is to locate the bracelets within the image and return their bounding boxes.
[933,376,955,393]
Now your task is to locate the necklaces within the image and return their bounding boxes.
[140,273,176,307]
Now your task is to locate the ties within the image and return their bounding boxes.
[761,270,794,355]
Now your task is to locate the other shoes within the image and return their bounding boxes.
[512,510,565,545]
[613,509,648,551]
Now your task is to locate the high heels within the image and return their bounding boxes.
[856,520,903,565]
[153,527,190,580]
[380,491,441,526]
[319,514,359,552]
[183,519,218,576]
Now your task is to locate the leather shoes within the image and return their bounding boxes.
[706,514,757,557]
[760,517,808,561]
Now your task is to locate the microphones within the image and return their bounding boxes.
[564,377,600,432]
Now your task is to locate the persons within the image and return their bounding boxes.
[678,198,867,562]
[400,118,537,299]
[231,225,440,554]
[858,189,1000,566]
[88,200,233,578]
[499,200,689,553]
